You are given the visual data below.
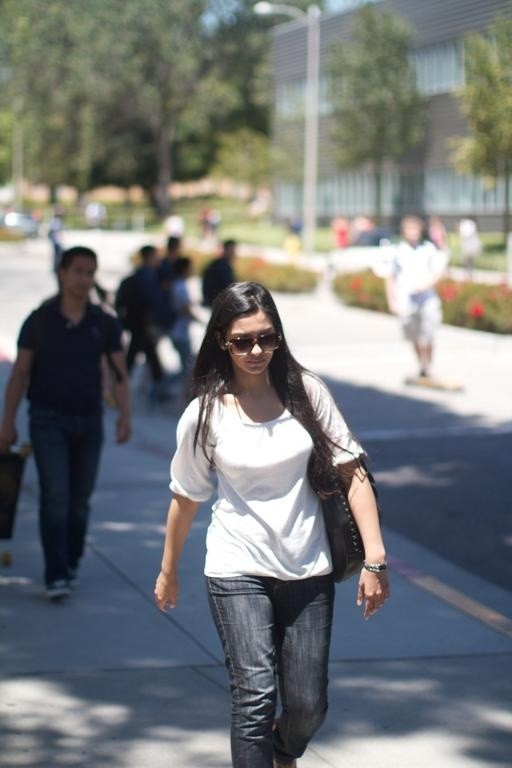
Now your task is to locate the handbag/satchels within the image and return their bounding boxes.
[295,366,381,582]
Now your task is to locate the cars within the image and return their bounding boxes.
[0,209,41,239]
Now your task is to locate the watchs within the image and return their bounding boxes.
[359,560,389,575]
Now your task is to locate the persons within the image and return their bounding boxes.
[112,234,238,406]
[0,242,136,602]
[379,213,448,380]
[0,349,37,572]
[2,201,486,276]
[150,278,393,766]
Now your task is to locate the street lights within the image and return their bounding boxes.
[247,1,322,257]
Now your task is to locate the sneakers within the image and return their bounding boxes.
[44,567,86,598]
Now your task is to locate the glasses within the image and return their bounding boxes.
[223,334,283,356]
